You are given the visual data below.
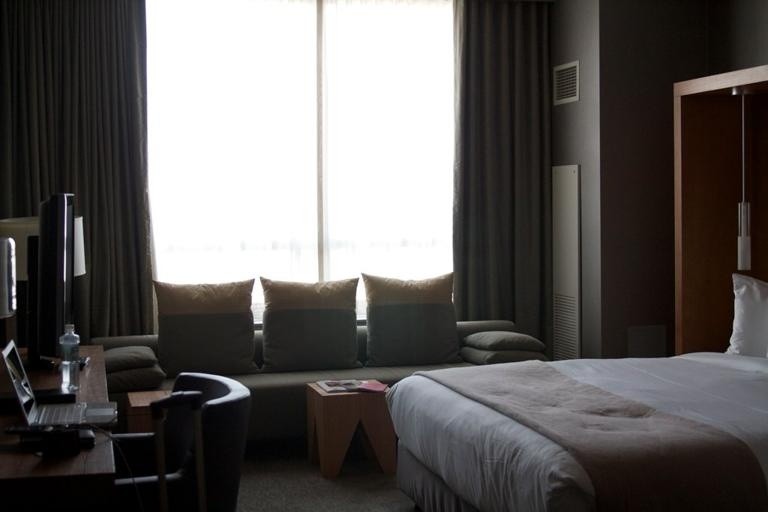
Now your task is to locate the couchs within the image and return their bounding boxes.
[90,319,552,442]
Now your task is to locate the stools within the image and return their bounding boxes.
[305,381,398,478]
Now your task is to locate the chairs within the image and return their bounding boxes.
[87,370,253,511]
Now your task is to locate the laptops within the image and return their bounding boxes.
[1,339,118,429]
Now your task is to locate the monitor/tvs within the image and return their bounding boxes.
[26,192,76,371]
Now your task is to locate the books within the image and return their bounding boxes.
[357,382,388,392]
[316,380,363,392]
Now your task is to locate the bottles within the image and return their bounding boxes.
[57,324,81,393]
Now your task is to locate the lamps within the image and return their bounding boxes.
[0,215,87,348]
[731,85,754,272]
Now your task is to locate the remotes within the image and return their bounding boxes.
[79,354,91,369]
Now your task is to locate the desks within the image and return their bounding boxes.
[1,344,119,511]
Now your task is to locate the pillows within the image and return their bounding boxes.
[106,362,166,394]
[105,345,156,373]
[151,279,261,379]
[260,278,363,375]
[462,331,548,351]
[362,272,464,366]
[459,346,552,366]
[723,272,768,357]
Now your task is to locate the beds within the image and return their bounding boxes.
[383,351,768,512]
